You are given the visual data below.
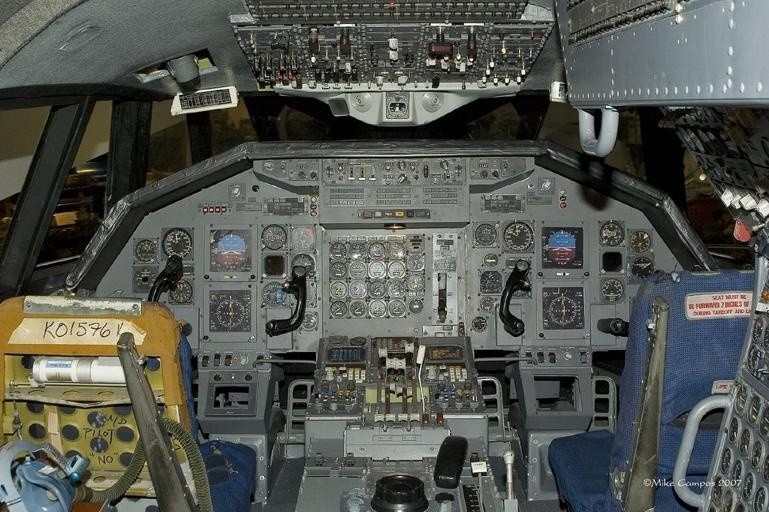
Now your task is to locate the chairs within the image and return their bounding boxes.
[0,295,256,512]
[548,272,756,511]
